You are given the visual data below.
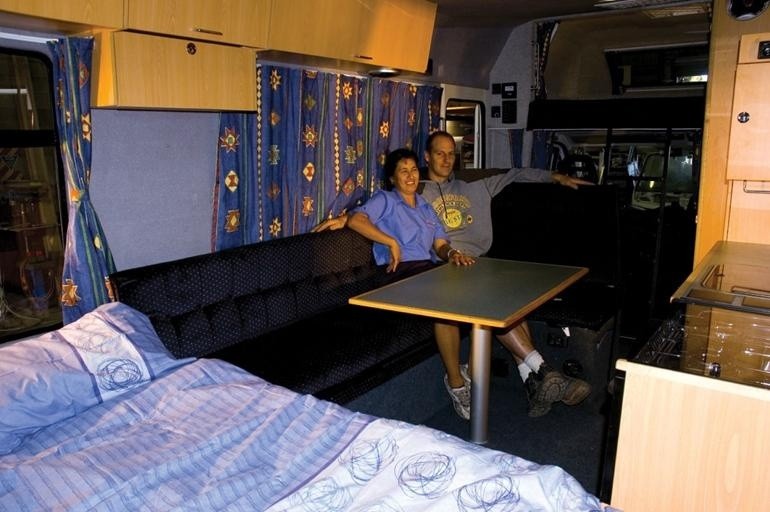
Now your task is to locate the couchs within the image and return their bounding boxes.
[111,183,634,426]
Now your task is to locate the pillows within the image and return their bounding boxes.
[0,302,196,455]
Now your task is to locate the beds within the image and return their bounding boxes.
[0,330,612,512]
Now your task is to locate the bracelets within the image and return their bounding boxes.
[447,248,461,259]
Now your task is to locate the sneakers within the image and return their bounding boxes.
[540,363,592,407]
[444,373,472,422]
[460,363,472,391]
[523,370,565,418]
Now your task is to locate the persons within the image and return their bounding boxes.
[310,130,596,421]
[347,148,476,420]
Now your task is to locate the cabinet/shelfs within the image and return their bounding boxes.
[0,0,438,114]
